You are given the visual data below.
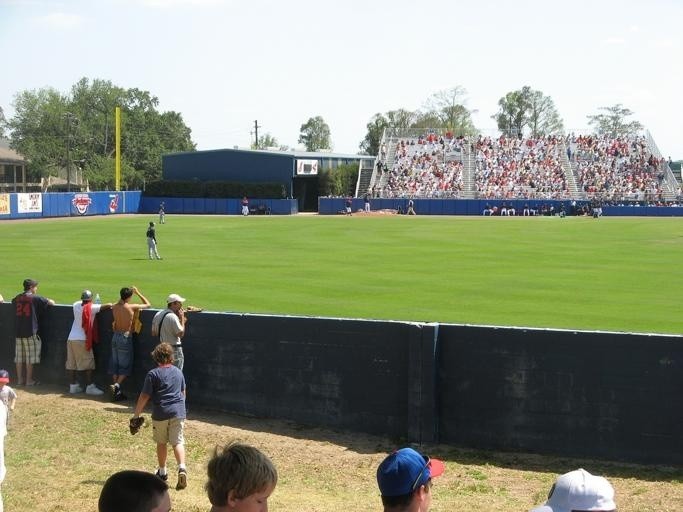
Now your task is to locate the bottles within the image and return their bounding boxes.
[95,294,101,304]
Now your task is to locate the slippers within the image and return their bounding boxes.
[24,380,41,386]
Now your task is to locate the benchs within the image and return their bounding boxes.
[481,211,555,216]
[387,138,675,200]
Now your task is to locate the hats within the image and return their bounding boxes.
[166,293,185,304]
[0,369,9,384]
[376,447,444,498]
[23,278,38,290]
[80,289,93,301]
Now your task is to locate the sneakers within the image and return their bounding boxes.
[176,468,186,489]
[69,383,127,402]
[154,469,168,482]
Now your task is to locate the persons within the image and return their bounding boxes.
[241,196,249,216]
[377,448,445,512]
[98,471,171,512]
[366,131,469,199]
[147,222,163,260]
[572,133,679,207]
[0,370,17,425]
[483,199,602,216]
[471,131,571,199]
[158,202,165,224]
[398,199,416,215]
[346,196,353,216]
[206,445,277,512]
[0,280,187,401]
[130,343,187,490]
[364,193,371,212]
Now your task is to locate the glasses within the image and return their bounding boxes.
[408,455,431,497]
